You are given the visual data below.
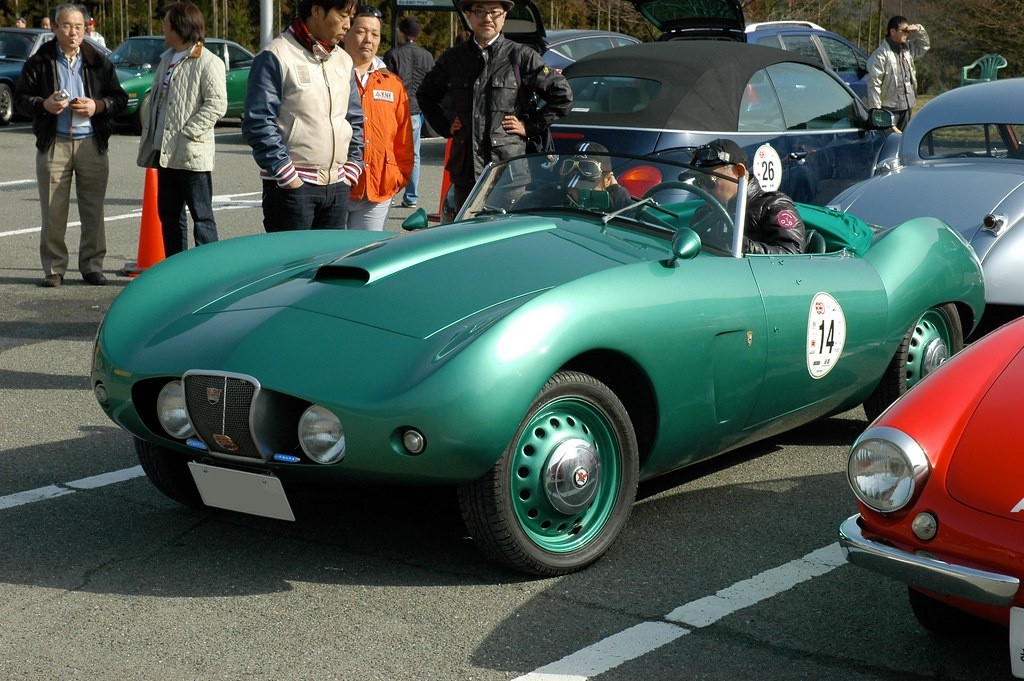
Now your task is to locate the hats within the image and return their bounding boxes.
[458,0,514,14]
[678,138,746,183]
[399,17,421,35]
[561,142,611,190]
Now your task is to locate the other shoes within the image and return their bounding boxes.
[43,274,63,287]
[402,199,416,208]
[83,272,107,285]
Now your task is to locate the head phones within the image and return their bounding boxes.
[885,35,909,52]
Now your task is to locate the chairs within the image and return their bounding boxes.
[960,52,1009,87]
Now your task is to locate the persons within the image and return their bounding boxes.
[16,17,106,48]
[384,18,436,208]
[241,0,365,232]
[867,15,930,133]
[136,0,227,258]
[678,138,806,255]
[454,31,471,45]
[416,0,573,213]
[338,4,415,232]
[513,141,632,218]
[18,4,129,287]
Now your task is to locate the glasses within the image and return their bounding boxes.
[689,147,723,166]
[560,160,603,180]
[472,9,506,18]
[692,174,720,189]
[56,21,86,33]
[355,6,383,18]
[897,29,909,33]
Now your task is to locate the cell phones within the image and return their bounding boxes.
[578,190,610,209]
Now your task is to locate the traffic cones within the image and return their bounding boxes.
[422,137,452,223]
[120,166,166,276]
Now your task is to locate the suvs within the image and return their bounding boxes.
[630,0,876,133]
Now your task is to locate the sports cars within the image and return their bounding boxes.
[88,153,984,575]
[838,312,1023,647]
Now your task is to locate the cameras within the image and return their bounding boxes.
[54,89,70,101]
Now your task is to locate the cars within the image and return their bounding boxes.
[106,36,258,134]
[818,74,1024,330]
[439,39,904,215]
[450,0,656,117]
[0,28,127,128]
